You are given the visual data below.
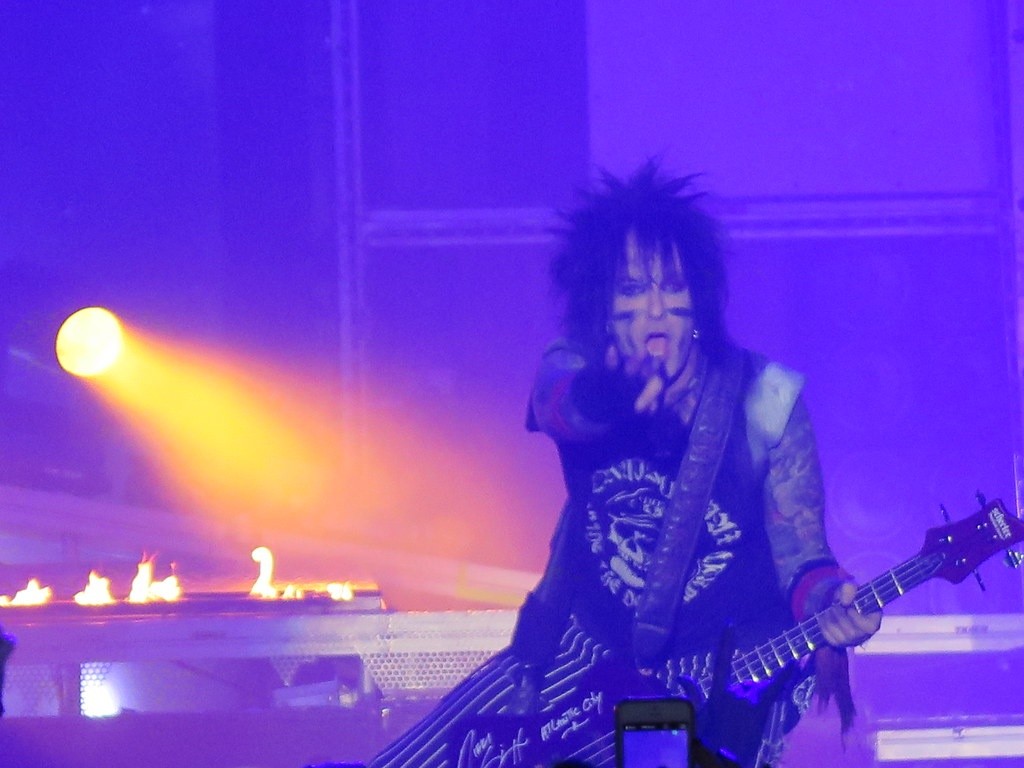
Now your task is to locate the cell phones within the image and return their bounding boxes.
[614,696,696,768]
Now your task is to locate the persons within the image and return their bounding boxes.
[367,153,882,768]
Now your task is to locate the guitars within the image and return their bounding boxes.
[443,486,1024,767]
